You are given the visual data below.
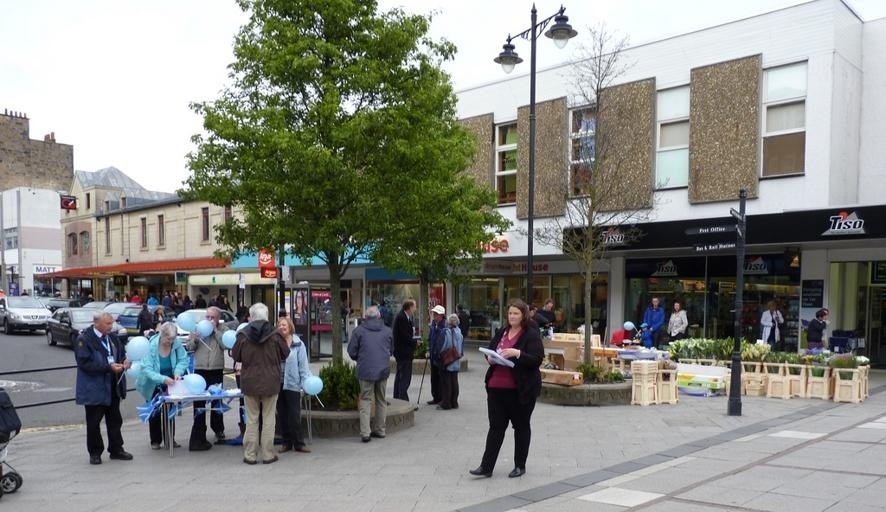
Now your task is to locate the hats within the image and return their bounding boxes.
[430,305,446,316]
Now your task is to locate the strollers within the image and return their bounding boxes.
[0,385,23,499]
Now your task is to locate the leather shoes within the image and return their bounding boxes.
[509,467,525,477]
[469,466,492,478]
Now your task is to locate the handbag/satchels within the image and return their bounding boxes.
[440,347,459,367]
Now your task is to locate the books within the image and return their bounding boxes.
[479,347,515,368]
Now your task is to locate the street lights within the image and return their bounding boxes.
[491,2,578,317]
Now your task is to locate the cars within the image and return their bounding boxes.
[0,294,177,351]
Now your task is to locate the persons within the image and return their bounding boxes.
[469,299,546,478]
[806,310,826,351]
[75,288,315,464]
[666,300,688,344]
[610,322,634,348]
[346,305,394,441]
[644,296,665,348]
[432,313,464,410]
[456,303,470,339]
[761,301,784,352]
[425,305,448,404]
[378,302,390,326]
[640,323,653,349]
[393,300,422,402]
[527,299,563,340]
[821,307,830,348]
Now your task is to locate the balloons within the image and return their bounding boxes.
[303,375,324,395]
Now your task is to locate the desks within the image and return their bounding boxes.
[160,386,312,457]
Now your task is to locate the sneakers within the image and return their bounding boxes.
[90,455,101,464]
[279,442,292,452]
[152,443,160,449]
[295,443,311,452]
[264,456,277,464]
[173,440,180,448]
[110,452,132,459]
[243,457,258,464]
[362,435,370,441]
[371,432,385,438]
[216,431,224,438]
[428,400,458,409]
